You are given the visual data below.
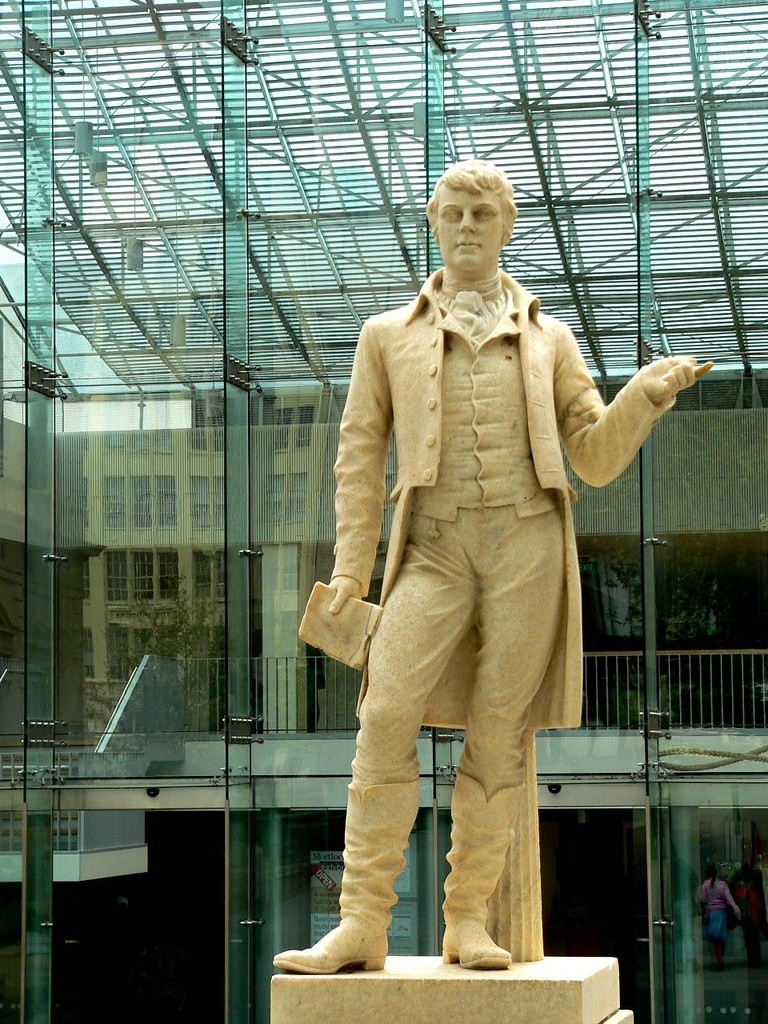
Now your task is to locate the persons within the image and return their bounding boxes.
[695,843,768,973]
[271,156,712,972]
